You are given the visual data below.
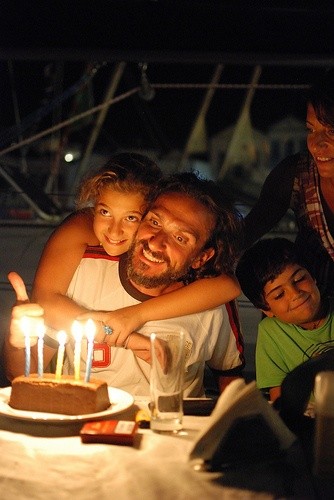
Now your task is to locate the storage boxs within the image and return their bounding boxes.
[80,420,138,448]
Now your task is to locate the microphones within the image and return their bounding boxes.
[138,63,156,102]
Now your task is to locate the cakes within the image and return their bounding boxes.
[9,372,110,415]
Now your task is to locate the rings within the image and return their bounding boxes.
[103,325,113,334]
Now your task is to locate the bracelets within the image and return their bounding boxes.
[124,337,131,349]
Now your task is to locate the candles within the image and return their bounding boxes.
[18,317,97,383]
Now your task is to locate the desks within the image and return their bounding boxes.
[0,398,278,500]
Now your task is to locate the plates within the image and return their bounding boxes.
[0,385,135,425]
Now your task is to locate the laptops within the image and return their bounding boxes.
[0,162,74,221]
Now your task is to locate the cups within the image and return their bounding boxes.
[149,332,187,433]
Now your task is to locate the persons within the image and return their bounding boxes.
[5,169,245,396]
[234,238,334,402]
[31,152,241,374]
[229,78,334,320]
